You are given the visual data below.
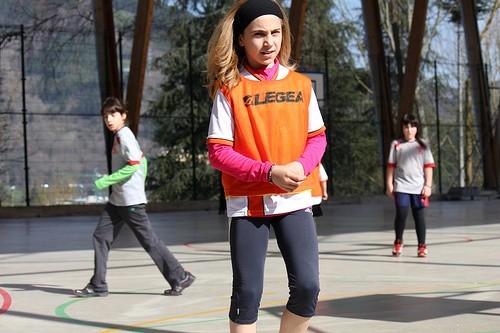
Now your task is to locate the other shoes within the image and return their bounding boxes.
[219,210,225,214]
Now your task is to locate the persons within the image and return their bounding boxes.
[71,96,197,298]
[312,164,328,223]
[385,114,437,257]
[202,0,328,333]
[217,171,226,215]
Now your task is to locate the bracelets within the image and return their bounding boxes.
[268,169,273,183]
[426,184,432,187]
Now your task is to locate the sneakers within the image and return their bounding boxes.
[418,243,428,257]
[72,283,108,298]
[164,272,195,297]
[392,239,404,257]
